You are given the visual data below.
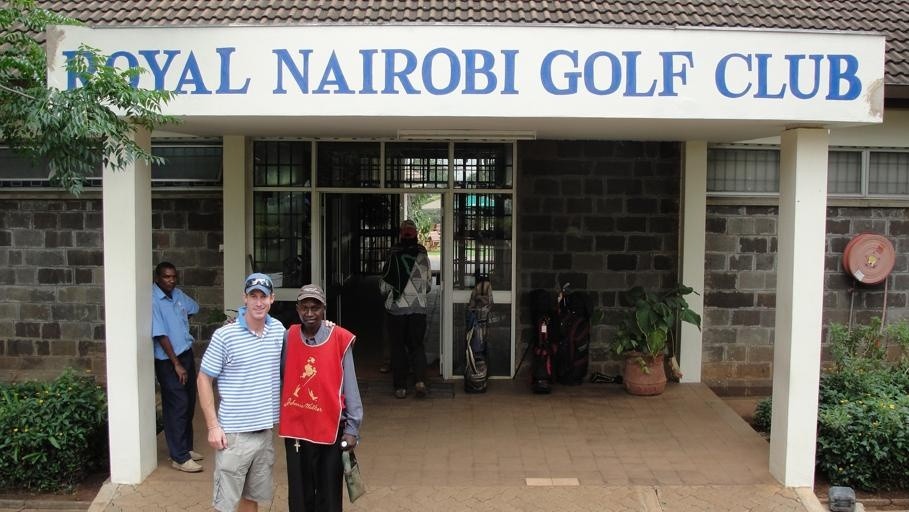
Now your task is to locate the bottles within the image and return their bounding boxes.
[341,434,360,448]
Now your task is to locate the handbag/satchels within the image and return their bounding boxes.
[343,448,367,504]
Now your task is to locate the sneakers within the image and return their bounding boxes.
[380,363,392,373]
[171,457,203,473]
[394,388,407,399]
[416,382,426,398]
[188,450,204,461]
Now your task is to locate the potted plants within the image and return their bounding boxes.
[615,283,701,395]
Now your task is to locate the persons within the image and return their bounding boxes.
[222,283,364,512]
[378,220,432,398]
[153,261,204,473]
[197,273,335,512]
[379,245,427,376]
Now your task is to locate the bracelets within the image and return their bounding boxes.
[207,425,219,430]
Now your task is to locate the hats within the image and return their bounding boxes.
[244,271,274,295]
[297,284,327,308]
[401,220,417,239]
